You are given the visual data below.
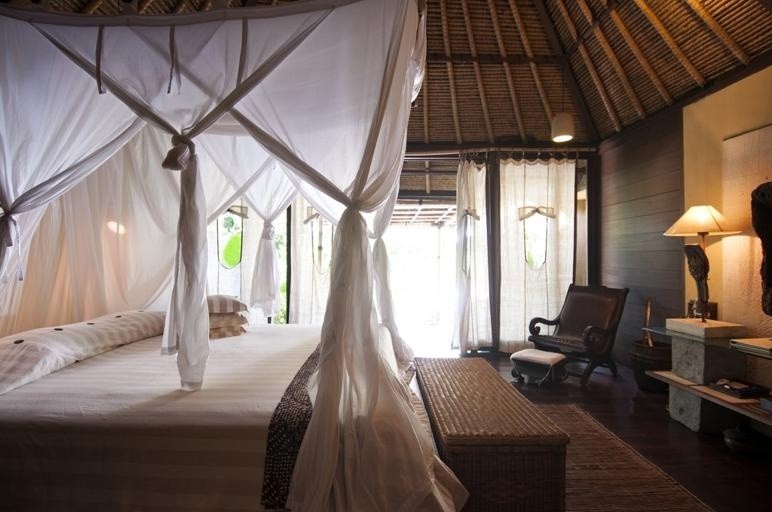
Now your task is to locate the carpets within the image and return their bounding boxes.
[532,401,716,512]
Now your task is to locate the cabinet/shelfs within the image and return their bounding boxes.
[639,326,772,427]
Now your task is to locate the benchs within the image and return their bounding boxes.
[414,355,571,512]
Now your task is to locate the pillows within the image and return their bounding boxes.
[0,295,247,397]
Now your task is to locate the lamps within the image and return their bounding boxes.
[661,204,743,322]
[551,63,576,143]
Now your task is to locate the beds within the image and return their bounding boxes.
[0,324,471,512]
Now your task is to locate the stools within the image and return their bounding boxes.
[509,349,570,390]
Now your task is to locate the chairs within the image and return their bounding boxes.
[527,283,629,386]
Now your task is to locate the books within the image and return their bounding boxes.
[730,336,772,355]
